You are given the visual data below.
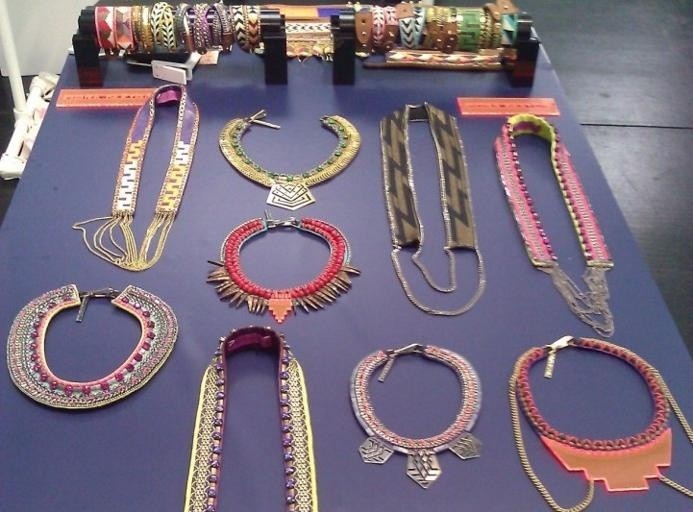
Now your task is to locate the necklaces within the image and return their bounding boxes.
[380,102,487,318]
[207,212,361,324]
[492,110,616,340]
[506,334,692,512]
[346,343,486,488]
[6,282,177,411]
[183,325,318,512]
[71,83,199,273]
[217,110,360,212]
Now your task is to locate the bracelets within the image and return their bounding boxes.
[96,4,258,54]
[355,1,500,53]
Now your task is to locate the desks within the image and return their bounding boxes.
[0,10,692,511]
